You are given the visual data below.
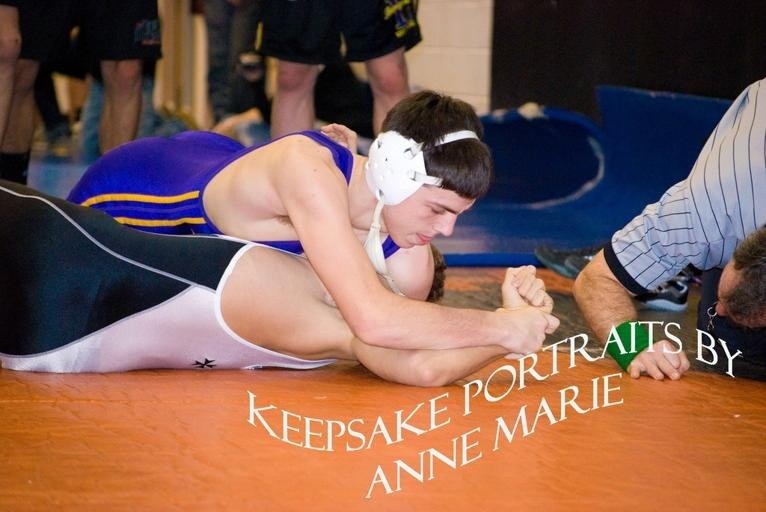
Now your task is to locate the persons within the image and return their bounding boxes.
[0,1,68,186]
[532,241,706,315]
[0,122,553,386]
[64,88,565,355]
[260,1,422,140]
[30,66,71,147]
[81,62,157,161]
[77,1,162,156]
[187,1,270,122]
[570,78,766,382]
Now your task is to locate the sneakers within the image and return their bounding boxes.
[629,270,690,312]
[535,244,601,281]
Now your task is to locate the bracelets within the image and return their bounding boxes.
[606,319,655,372]
